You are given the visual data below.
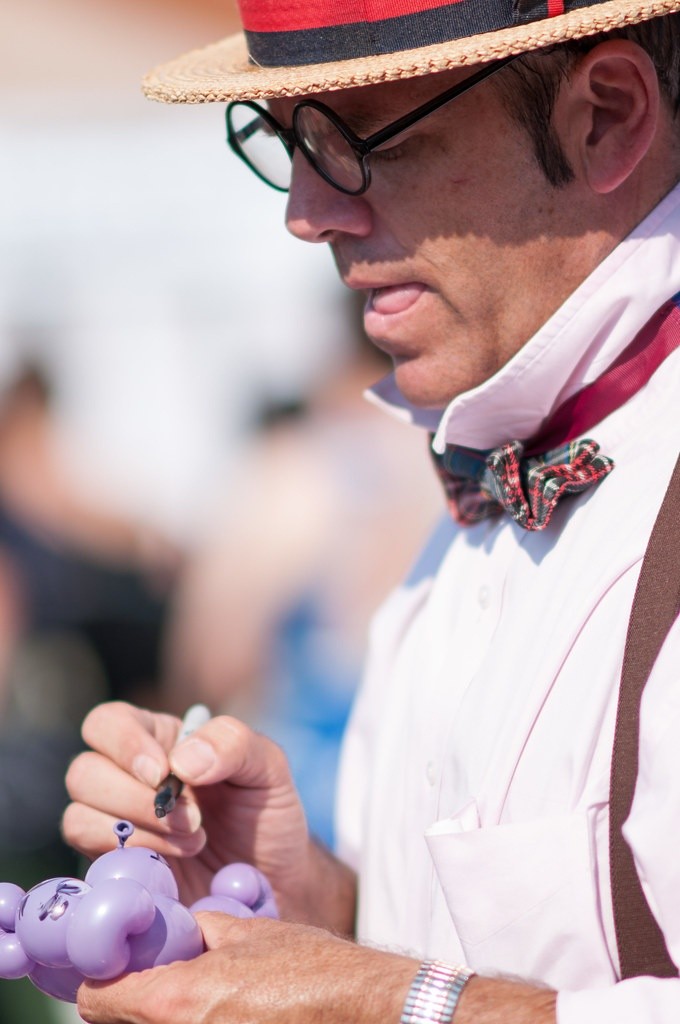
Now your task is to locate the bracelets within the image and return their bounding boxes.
[399,959,475,1024]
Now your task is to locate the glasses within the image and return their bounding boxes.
[225,48,527,194]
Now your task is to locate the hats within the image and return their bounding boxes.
[141,1,679,105]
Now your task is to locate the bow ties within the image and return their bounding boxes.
[424,297,680,533]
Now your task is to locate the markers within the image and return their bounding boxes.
[154,703,211,821]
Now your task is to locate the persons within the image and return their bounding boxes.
[61,0,680,1024]
